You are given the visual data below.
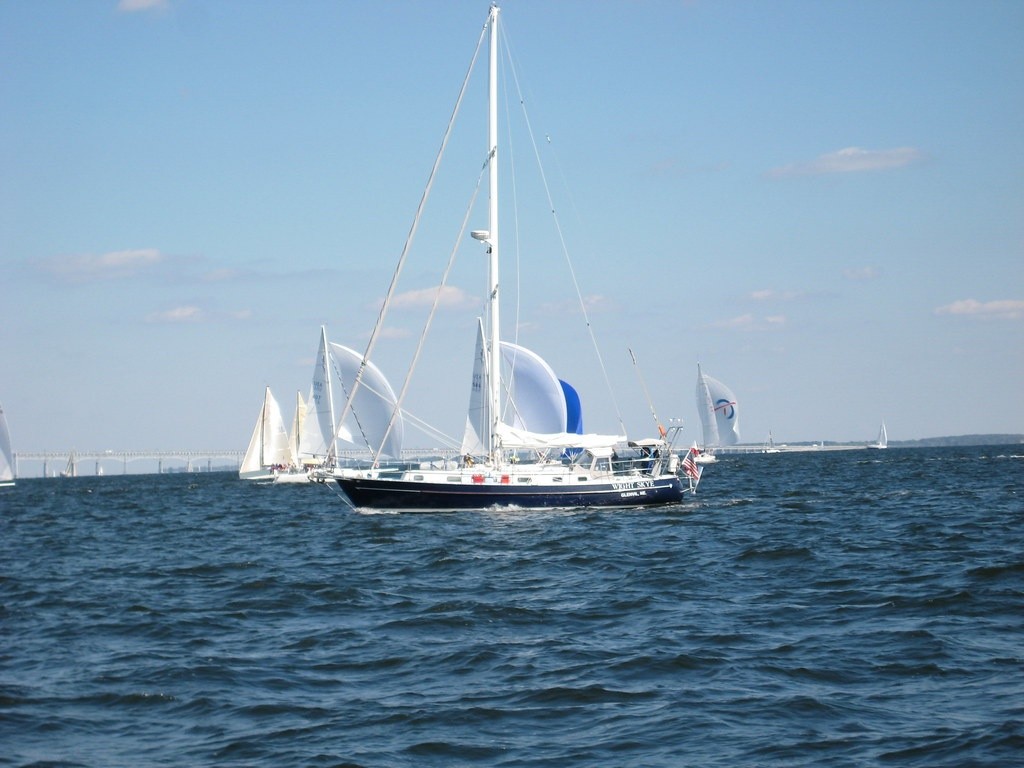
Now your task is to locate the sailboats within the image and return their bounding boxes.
[236,385,339,483]
[303,1,707,506]
[691,359,739,464]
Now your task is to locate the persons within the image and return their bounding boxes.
[642,448,652,475]
[508,450,515,463]
[463,453,472,468]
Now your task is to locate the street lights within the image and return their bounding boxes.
[867,420,888,449]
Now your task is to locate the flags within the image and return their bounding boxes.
[682,447,699,481]
